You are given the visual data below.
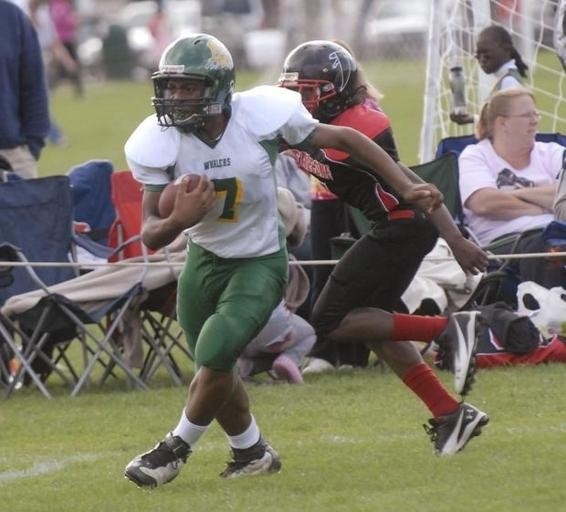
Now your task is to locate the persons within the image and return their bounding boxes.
[450,25,529,142]
[457,88,566,312]
[0,0,51,180]
[124,34,445,492]
[234,185,317,385]
[32,0,83,98]
[278,40,491,457]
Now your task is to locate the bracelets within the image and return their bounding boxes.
[540,207,545,214]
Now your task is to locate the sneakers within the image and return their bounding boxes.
[273,355,304,384]
[219,435,282,478]
[436,311,481,396]
[125,432,193,487]
[428,401,489,456]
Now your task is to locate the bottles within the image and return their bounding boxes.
[448,65,468,117]
[5,344,26,386]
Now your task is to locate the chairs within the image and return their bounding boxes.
[329,129,566,365]
[67,158,183,394]
[0,174,149,396]
[108,166,195,383]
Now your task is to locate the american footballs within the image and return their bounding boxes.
[157,173,206,228]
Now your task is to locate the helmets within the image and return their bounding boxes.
[279,40,357,124]
[152,33,234,127]
